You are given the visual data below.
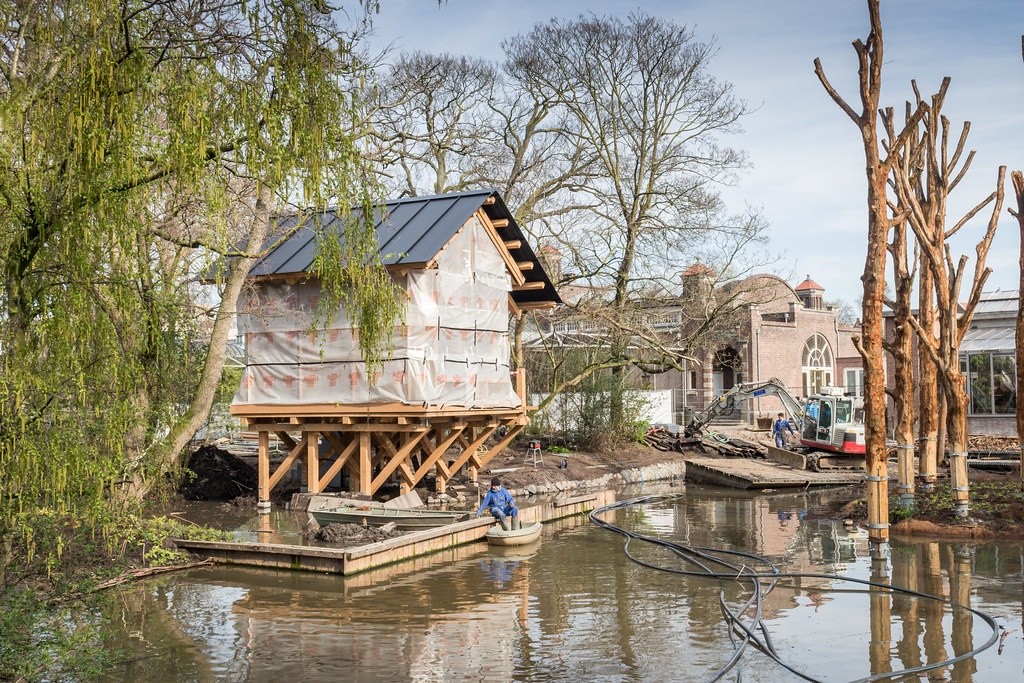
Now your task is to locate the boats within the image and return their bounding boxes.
[487,535,542,564]
[484,519,543,547]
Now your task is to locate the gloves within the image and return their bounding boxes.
[772,434,775,439]
[509,503,514,508]
[792,433,796,438]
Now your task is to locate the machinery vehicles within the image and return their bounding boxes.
[674,375,866,474]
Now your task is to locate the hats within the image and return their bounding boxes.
[491,477,501,486]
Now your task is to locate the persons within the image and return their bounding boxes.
[477,558,518,590]
[475,477,518,530]
[815,404,831,427]
[773,413,797,448]
[778,509,792,528]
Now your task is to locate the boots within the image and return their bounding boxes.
[500,521,509,531]
[510,517,519,531]
